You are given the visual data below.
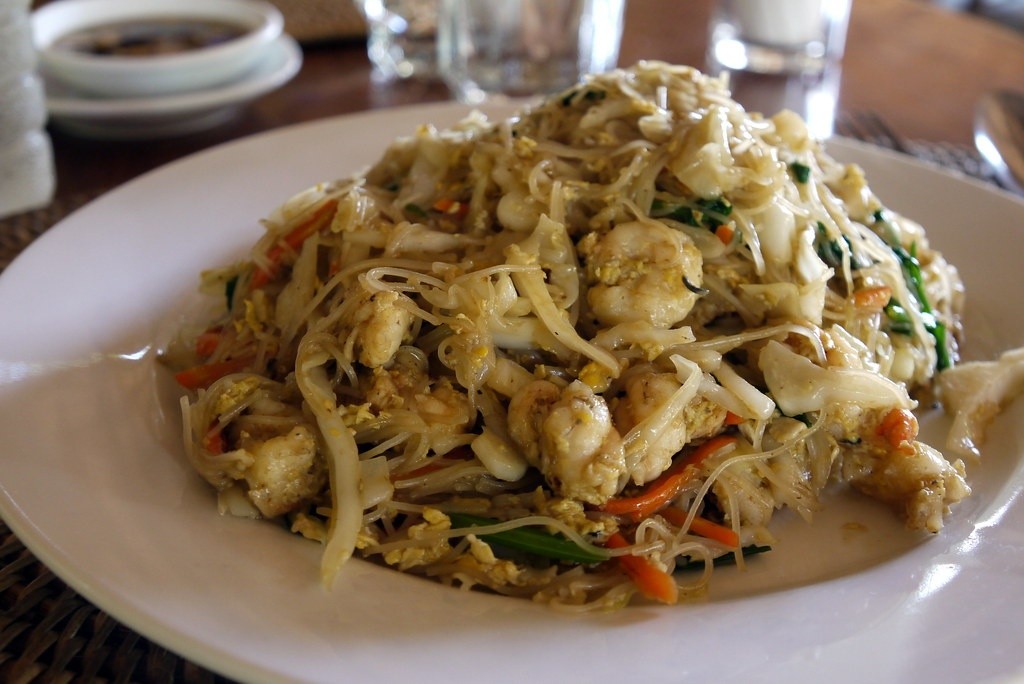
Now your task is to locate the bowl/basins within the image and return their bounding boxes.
[32,0,282,87]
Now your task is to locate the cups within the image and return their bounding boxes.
[0,0,51,210]
[442,0,625,104]
[703,0,852,145]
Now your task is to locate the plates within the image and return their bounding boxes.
[34,35,301,142]
[1,100,1024,684]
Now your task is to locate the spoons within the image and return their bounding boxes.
[972,89,1024,195]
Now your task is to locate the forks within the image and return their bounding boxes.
[836,111,916,153]
